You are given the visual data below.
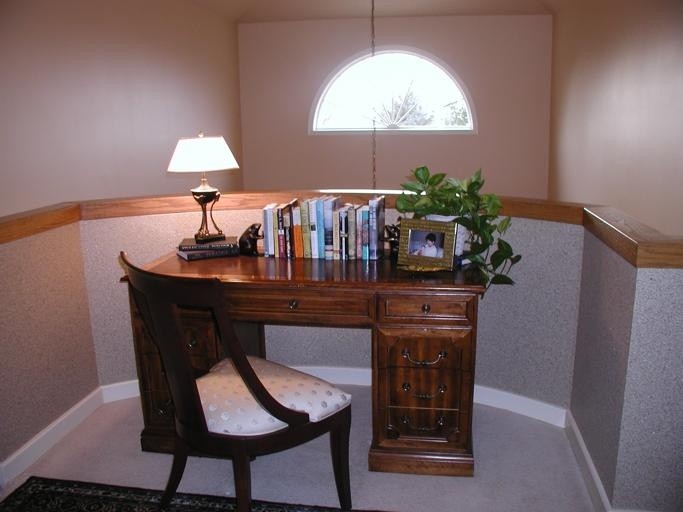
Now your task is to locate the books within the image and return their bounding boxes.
[263,193,386,260]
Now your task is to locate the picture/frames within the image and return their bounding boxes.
[396,218,458,271]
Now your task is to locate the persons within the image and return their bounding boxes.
[415,234,438,258]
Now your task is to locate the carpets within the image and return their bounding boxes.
[0,473,374,512]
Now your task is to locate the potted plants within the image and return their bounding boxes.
[393,164,523,300]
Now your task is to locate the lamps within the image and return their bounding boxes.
[167,134,239,242]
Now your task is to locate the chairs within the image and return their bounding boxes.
[121,253,351,512]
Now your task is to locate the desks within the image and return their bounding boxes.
[124,240,486,480]
